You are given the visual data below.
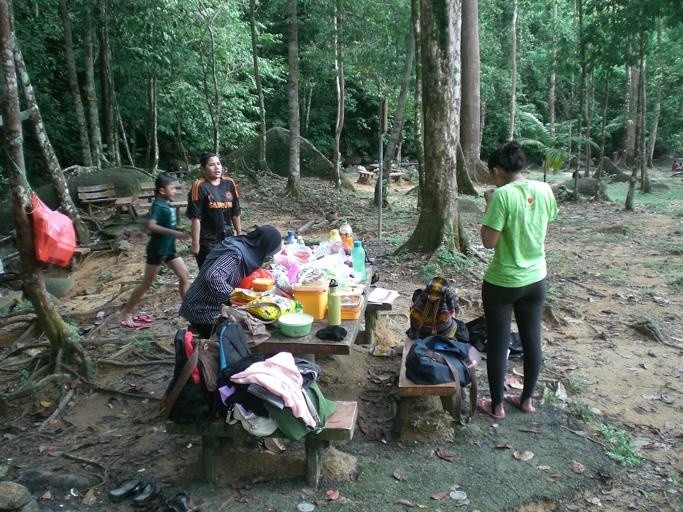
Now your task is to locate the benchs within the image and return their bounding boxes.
[357,160,401,186]
[76,180,193,227]
[164,396,368,490]
[388,332,461,438]
[364,286,396,341]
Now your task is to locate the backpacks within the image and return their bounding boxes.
[164,329,219,423]
[409,277,459,339]
[213,319,252,378]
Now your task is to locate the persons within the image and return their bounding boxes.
[186,153,241,271]
[671,160,683,170]
[119,173,190,327]
[179,225,282,333]
[477,140,560,419]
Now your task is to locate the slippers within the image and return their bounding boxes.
[121,320,143,329]
[504,394,528,412]
[478,397,506,419]
[133,315,153,323]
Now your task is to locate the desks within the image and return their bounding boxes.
[209,269,375,383]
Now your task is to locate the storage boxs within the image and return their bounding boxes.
[292,277,330,320]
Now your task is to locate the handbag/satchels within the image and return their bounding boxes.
[405,336,479,425]
[225,403,279,439]
[218,303,282,347]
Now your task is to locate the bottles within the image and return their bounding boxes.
[340,219,353,253]
[288,230,303,244]
[330,228,341,245]
[351,239,366,282]
[328,277,341,327]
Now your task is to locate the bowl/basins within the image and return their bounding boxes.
[278,313,315,338]
